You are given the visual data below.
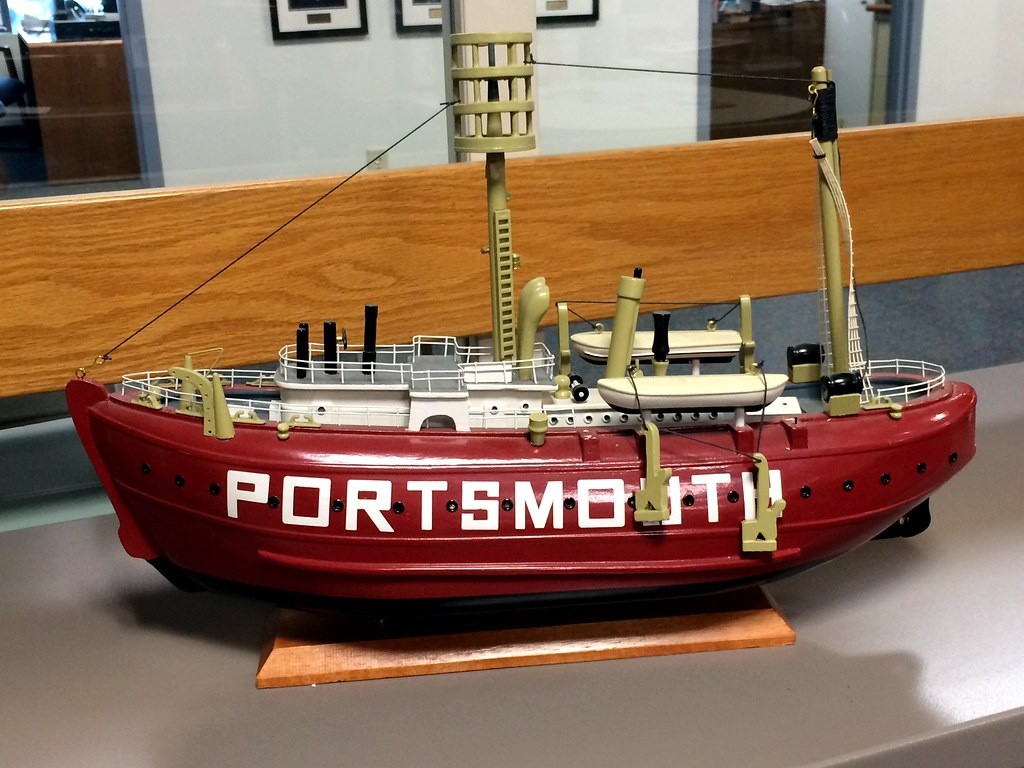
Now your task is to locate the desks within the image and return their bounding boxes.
[709,86,814,140]
[712,35,754,85]
[16,28,143,185]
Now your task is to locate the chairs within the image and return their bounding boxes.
[0,46,35,142]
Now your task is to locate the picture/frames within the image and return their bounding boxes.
[395,0,443,33]
[270,0,369,41]
[536,0,600,24]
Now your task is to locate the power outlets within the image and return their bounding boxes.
[367,150,387,169]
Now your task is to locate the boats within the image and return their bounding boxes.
[61,1,979,609]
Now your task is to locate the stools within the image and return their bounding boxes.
[743,60,803,93]
